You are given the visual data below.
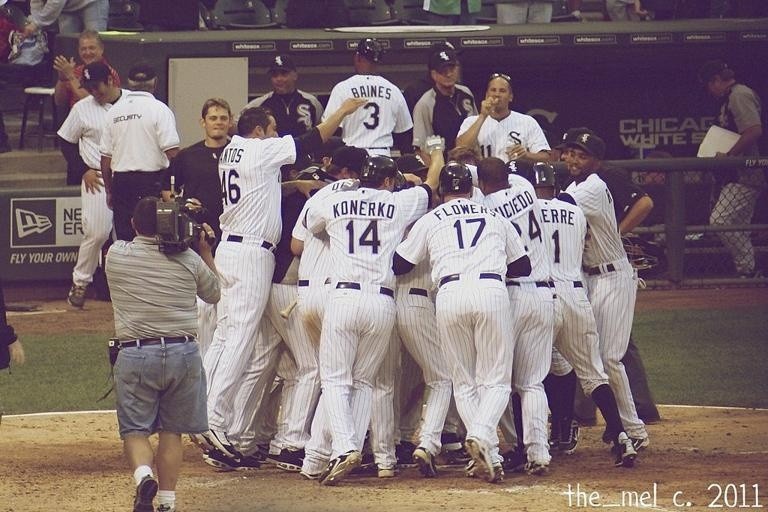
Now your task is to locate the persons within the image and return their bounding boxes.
[701,61,767,279]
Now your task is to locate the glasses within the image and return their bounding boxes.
[487,70,512,83]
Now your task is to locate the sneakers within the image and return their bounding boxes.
[67,282,86,308]
[189,417,661,487]
[132,476,158,512]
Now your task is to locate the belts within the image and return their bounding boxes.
[407,287,428,298]
[118,336,196,350]
[296,278,395,300]
[216,234,278,256]
[439,263,616,288]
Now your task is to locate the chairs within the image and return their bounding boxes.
[107,0,609,35]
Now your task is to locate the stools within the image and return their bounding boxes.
[20,87,60,153]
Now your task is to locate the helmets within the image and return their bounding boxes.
[358,155,398,186]
[440,163,473,196]
[530,162,557,188]
[355,37,384,64]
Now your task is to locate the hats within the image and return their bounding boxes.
[429,48,458,70]
[395,153,429,174]
[127,63,158,81]
[504,158,531,179]
[77,62,111,89]
[314,136,368,175]
[267,53,293,73]
[555,126,604,158]
[695,58,730,86]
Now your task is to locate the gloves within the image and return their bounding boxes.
[424,133,446,155]
